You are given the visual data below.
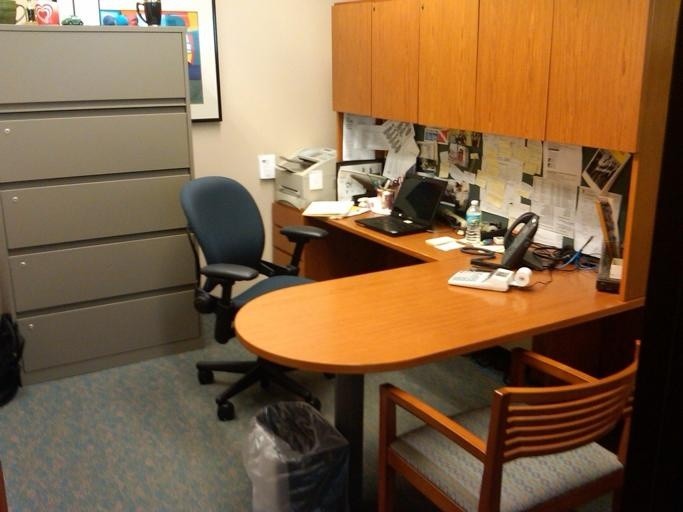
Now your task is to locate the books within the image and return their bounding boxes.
[301,200,353,219]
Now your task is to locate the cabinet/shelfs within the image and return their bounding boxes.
[0,25,206,388]
[330,0,681,154]
[273,201,366,281]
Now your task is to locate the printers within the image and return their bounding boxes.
[276,145,336,212]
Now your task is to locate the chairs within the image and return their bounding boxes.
[180,176,336,420]
[376,338,643,512]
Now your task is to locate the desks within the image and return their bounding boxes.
[231,200,648,512]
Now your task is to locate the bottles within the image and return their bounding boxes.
[465,201,481,245]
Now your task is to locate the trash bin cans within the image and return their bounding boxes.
[243,400,350,511]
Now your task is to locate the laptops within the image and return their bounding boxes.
[355,174,447,237]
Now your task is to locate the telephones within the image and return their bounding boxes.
[500,211,544,270]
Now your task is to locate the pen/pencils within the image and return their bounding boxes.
[372,178,399,190]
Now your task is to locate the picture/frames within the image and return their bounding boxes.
[72,0,222,123]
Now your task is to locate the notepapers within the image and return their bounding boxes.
[497,140,513,160]
[476,169,487,187]
[484,148,497,167]
[486,193,505,207]
[518,188,530,200]
[521,160,536,175]
[308,169,324,192]
[469,153,479,160]
[514,142,528,162]
[438,161,449,179]
[490,181,506,193]
[526,143,539,162]
[463,171,475,184]
